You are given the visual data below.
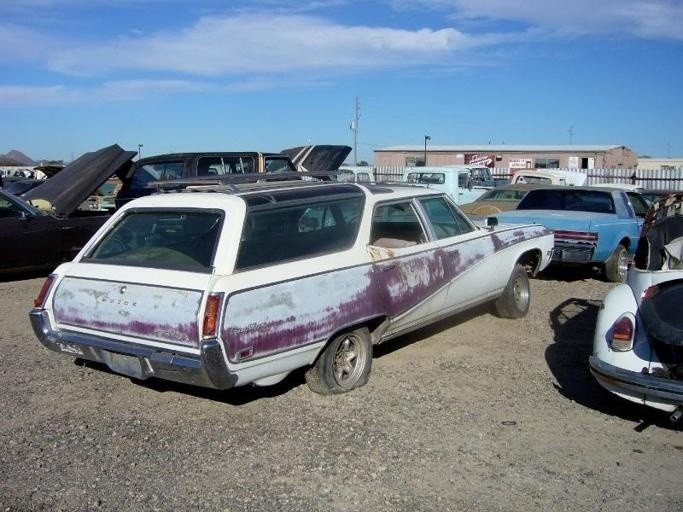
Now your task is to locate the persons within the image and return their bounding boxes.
[26,171,36,179]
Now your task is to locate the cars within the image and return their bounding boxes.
[28,169,555,395]
[589,191,682,425]
[0,143,383,281]
[403,166,682,282]
